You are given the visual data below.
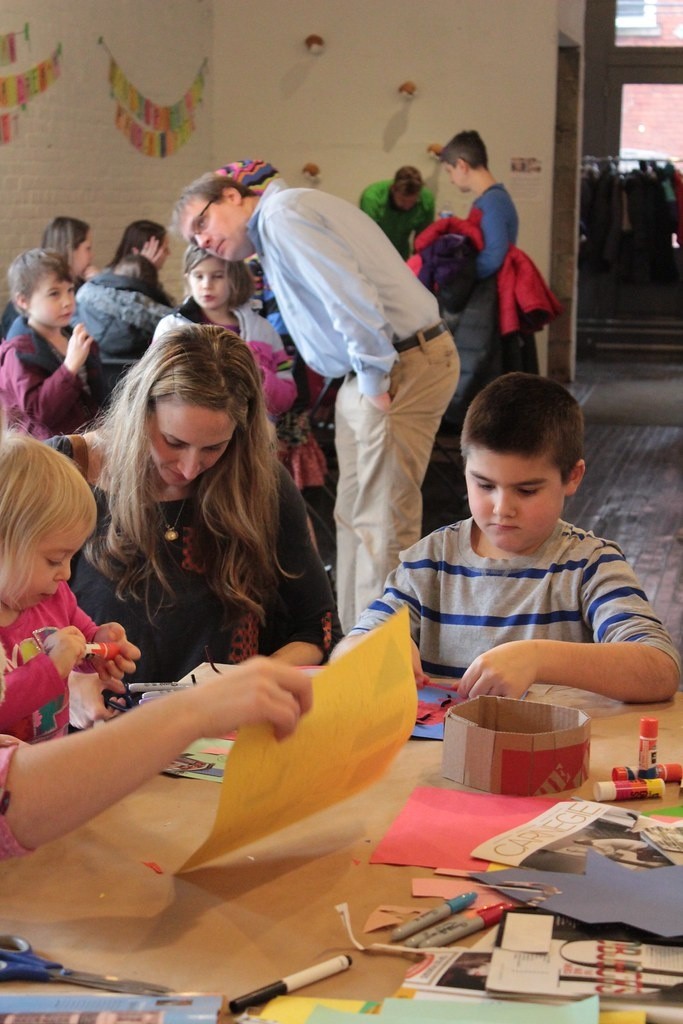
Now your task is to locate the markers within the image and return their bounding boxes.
[228,954,354,1013]
[390,891,513,950]
[128,681,194,704]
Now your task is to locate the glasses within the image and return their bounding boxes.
[189,196,219,246]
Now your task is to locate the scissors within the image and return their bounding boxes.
[0,933,174,997]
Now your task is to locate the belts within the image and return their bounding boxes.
[393,321,446,354]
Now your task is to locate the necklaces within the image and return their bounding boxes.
[153,485,192,540]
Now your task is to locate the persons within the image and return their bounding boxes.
[330,372,682,703]
[0,655,312,865]
[0,130,539,745]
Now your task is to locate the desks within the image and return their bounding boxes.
[0,678,683,1024]
